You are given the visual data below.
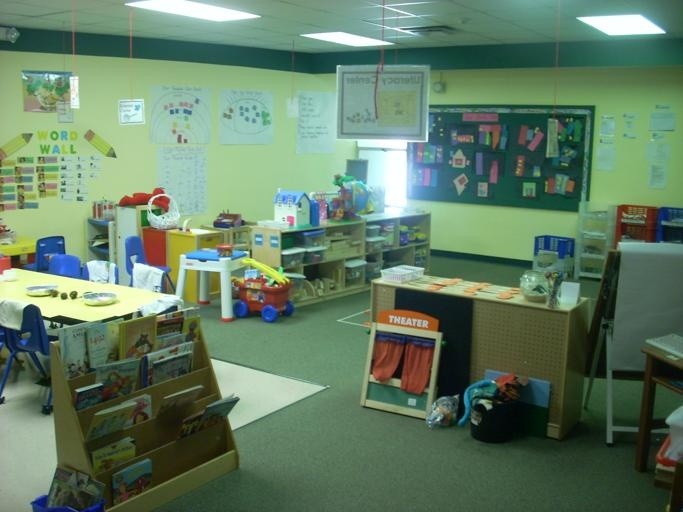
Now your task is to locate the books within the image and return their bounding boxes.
[46,306,239,512]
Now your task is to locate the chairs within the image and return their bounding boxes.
[20,235,66,271]
[126,234,175,295]
[48,254,81,279]
[127,262,165,322]
[83,259,120,285]
[1,300,70,415]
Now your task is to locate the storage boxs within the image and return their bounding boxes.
[281,223,384,288]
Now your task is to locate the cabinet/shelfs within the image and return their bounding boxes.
[46,320,243,510]
[165,223,251,307]
[356,206,430,290]
[249,204,363,310]
[86,215,116,264]
[527,202,683,285]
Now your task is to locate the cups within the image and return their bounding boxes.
[215,244,233,257]
[555,281,580,309]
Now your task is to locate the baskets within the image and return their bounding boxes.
[147,194,180,230]
[382,265,425,283]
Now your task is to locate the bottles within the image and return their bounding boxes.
[520,270,549,302]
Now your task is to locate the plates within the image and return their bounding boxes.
[26,285,56,297]
[81,291,117,306]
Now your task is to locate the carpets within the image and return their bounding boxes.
[1,348,330,511]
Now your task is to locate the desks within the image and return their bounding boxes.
[368,275,590,440]
[3,268,179,326]
[633,343,682,474]
[2,238,42,269]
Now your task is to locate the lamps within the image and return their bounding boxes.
[0,24,19,43]
